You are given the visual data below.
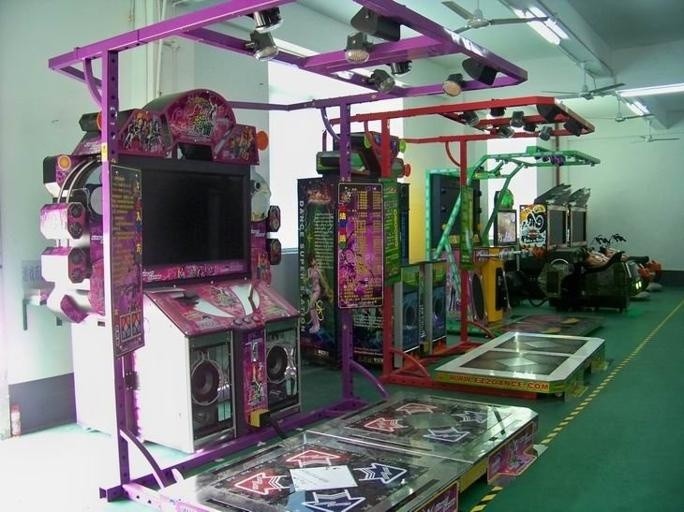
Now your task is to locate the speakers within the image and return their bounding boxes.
[468,274,486,320]
[189,336,231,435]
[264,325,300,406]
[428,287,448,336]
[401,291,422,349]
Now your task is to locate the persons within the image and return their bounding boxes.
[307,253,327,334]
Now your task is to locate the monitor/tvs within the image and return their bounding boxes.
[547,204,568,249]
[136,158,251,284]
[571,206,588,245]
[492,208,518,247]
